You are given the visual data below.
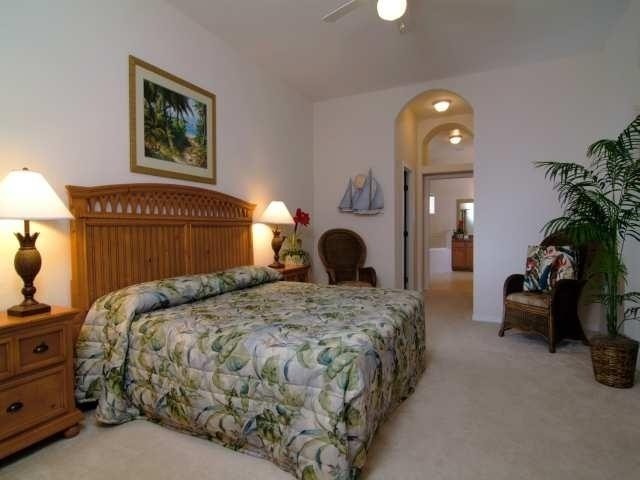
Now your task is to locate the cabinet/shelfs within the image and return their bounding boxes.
[451,234,474,273]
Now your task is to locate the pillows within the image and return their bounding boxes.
[522,240,580,295]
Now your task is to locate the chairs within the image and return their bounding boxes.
[498,226,595,356]
[316,226,379,290]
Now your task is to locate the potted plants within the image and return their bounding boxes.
[528,110,640,392]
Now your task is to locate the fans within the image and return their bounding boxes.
[321,0,412,35]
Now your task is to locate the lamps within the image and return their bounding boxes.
[254,195,297,271]
[374,0,409,24]
[0,164,78,319]
[448,133,463,145]
[431,98,452,116]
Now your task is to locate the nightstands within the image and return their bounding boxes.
[0,301,86,466]
[263,259,313,285]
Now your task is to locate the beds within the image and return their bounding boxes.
[59,180,433,480]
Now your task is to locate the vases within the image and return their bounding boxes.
[281,252,310,270]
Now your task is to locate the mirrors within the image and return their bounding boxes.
[455,197,473,238]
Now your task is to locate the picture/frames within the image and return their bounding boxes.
[123,52,218,188]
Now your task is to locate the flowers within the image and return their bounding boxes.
[276,205,313,264]
